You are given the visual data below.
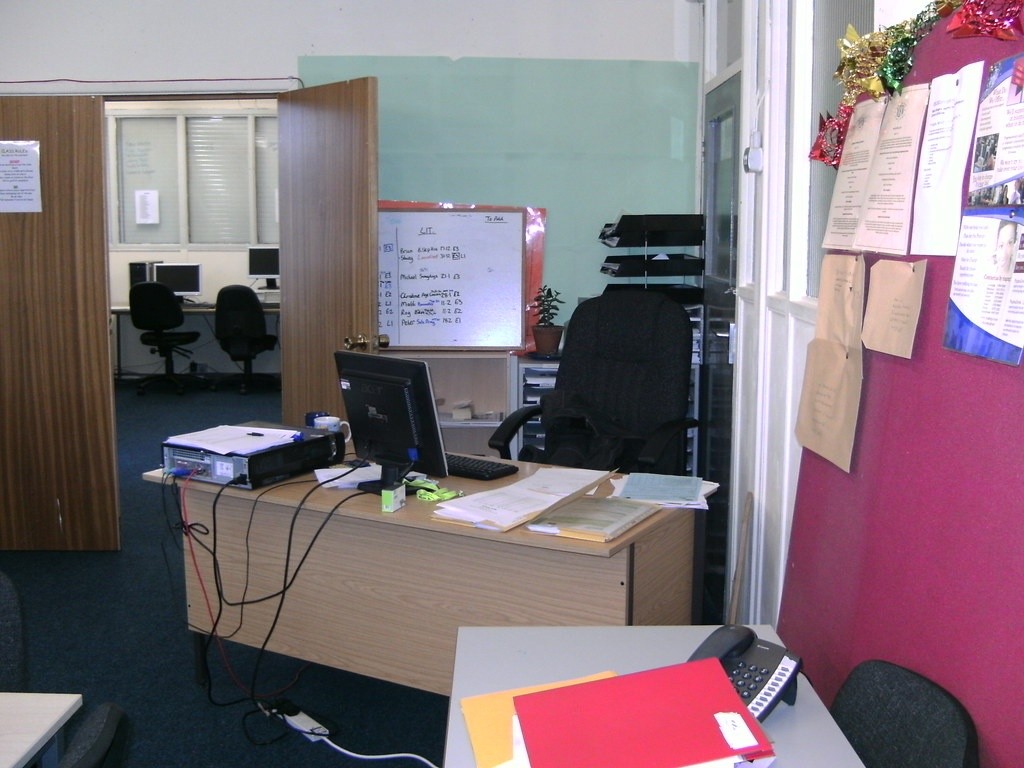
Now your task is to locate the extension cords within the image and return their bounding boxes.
[272,706,330,742]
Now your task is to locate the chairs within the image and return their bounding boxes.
[205,284,279,397]
[128,281,209,393]
[828,658,980,768]
[70,705,129,768]
[488,295,700,477]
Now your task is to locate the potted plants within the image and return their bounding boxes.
[531,285,565,353]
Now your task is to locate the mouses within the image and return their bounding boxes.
[209,303,217,309]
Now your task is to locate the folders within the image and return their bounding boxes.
[461,670,617,768]
[513,656,775,768]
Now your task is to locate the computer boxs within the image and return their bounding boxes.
[161,419,347,490]
[129,260,163,286]
[255,290,281,304]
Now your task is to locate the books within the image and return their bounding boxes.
[524,494,664,543]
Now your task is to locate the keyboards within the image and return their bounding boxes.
[179,303,209,309]
[445,453,519,480]
[261,303,281,309]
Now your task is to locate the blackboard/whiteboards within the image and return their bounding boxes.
[378,206,527,350]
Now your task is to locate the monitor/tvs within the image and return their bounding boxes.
[334,348,448,496]
[153,264,202,304]
[248,247,280,289]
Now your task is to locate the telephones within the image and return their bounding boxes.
[687,624,802,724]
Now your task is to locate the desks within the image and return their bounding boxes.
[141,464,720,699]
[0,687,83,768]
[112,302,281,384]
[441,624,867,768]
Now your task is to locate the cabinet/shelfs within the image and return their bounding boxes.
[379,352,520,461]
[520,357,699,475]
[601,208,705,304]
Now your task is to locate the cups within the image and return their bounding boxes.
[314,416,351,443]
[304,411,331,429]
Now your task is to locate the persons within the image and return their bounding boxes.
[994,219,1018,273]
[966,179,1021,206]
